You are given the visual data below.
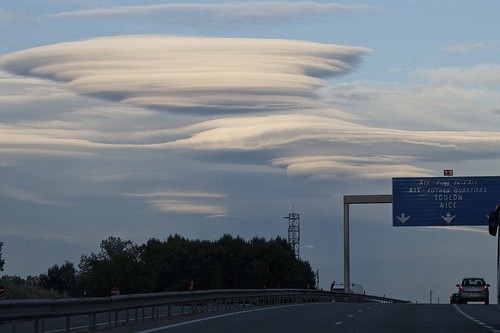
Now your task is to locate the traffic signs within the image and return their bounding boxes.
[391,176,499,226]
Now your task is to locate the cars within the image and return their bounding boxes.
[449,293,467,304]
[456,277,490,305]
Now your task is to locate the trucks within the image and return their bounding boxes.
[331,283,365,302]
